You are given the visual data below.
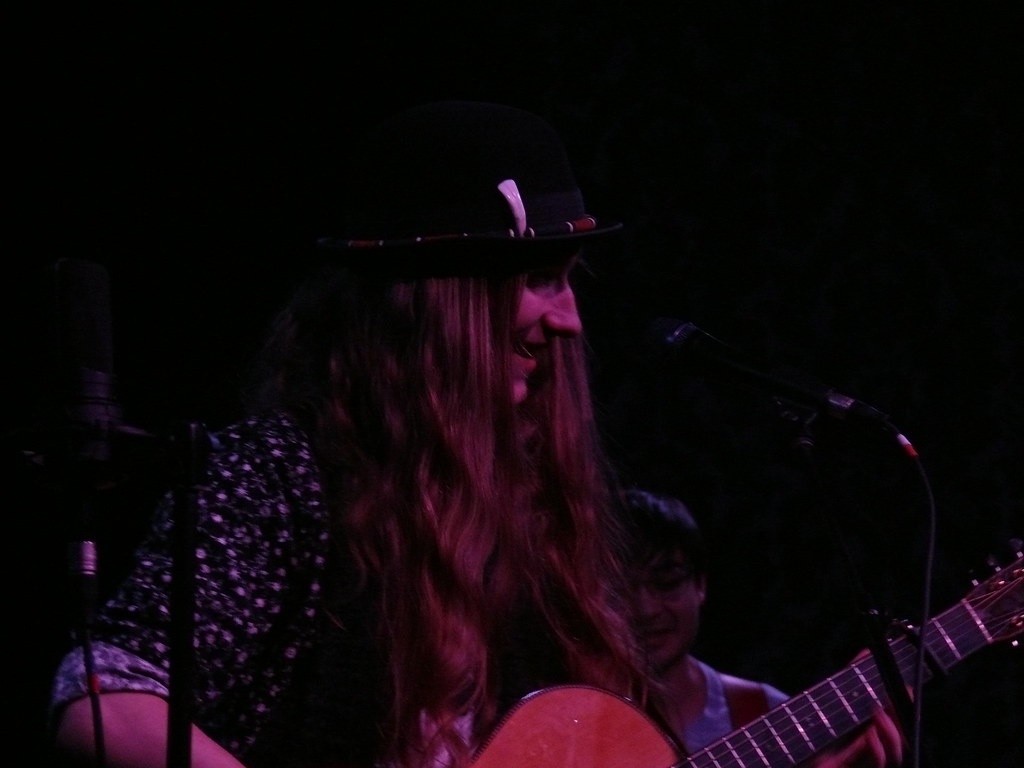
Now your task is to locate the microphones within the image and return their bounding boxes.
[646,314,891,430]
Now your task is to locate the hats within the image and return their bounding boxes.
[318,101,625,251]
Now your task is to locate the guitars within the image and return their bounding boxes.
[470,547,1024,767]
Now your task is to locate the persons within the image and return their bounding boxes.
[42,101,914,768]
